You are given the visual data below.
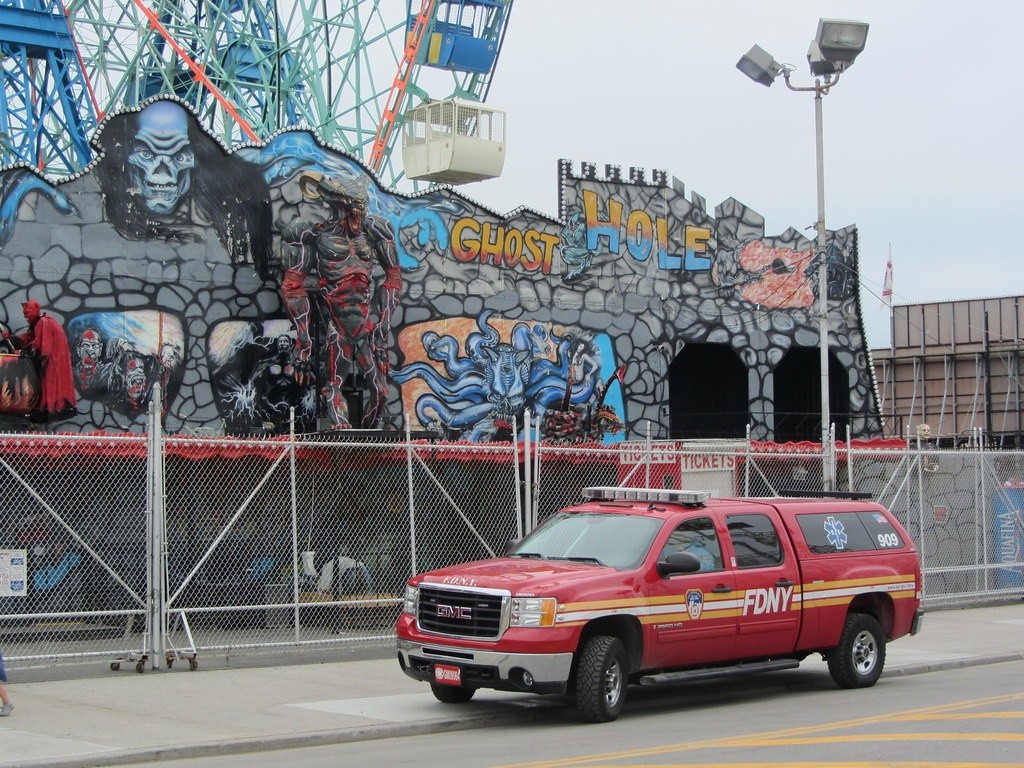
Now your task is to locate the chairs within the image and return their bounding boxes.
[330,567,372,629]
[22,553,284,635]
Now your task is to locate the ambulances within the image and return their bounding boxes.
[396,486,924,724]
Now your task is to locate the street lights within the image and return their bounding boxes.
[736,16,870,500]
